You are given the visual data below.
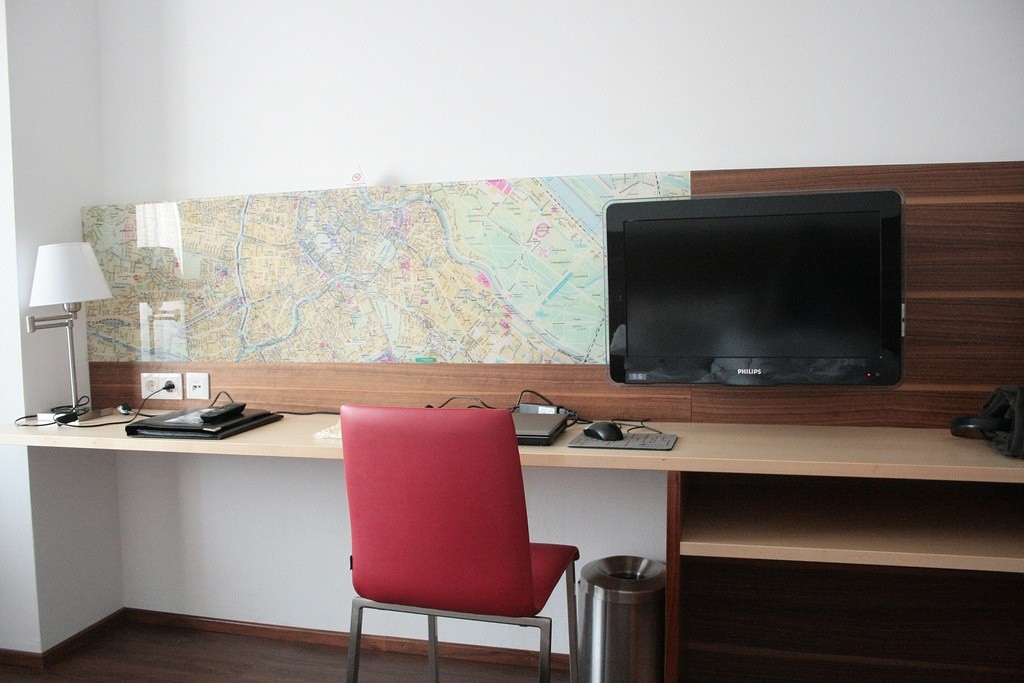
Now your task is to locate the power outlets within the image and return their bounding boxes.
[186,373,210,400]
[141,372,183,400]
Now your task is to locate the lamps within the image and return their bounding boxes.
[27,242,111,422]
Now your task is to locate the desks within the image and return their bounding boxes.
[1,407,1024,683]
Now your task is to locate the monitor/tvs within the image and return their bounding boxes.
[602,186,907,388]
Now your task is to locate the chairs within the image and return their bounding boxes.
[339,406,580,683]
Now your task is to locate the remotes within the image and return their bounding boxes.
[200,402,246,424]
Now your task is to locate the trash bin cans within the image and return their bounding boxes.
[572,554,666,683]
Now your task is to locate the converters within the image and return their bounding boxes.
[518,404,561,415]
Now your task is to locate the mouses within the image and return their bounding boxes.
[583,422,624,442]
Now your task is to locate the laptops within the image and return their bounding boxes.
[512,412,568,445]
